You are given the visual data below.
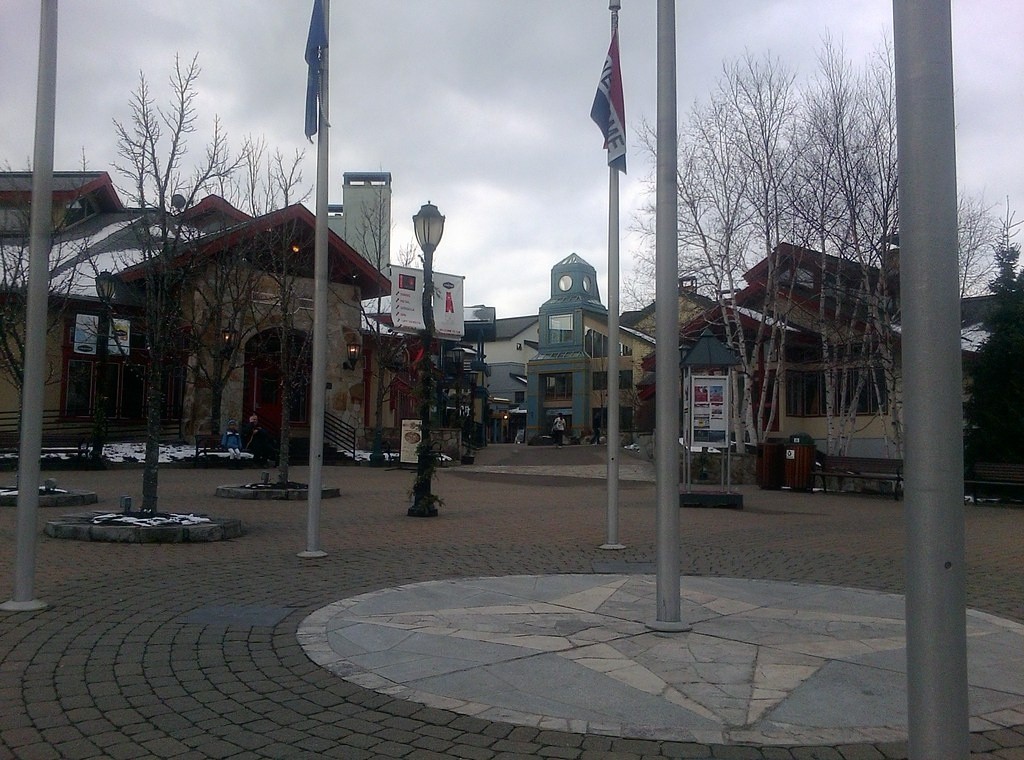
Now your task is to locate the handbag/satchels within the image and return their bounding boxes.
[551,424,558,432]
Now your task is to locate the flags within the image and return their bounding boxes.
[589,31,627,174]
[305,0,328,144]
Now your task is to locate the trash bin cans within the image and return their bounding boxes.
[755,444,785,490]
[789,432,815,446]
[782,444,817,492]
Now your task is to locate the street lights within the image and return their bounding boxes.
[407,200,446,516]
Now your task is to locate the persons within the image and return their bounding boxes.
[222,415,268,468]
[553,413,566,448]
[590,412,602,444]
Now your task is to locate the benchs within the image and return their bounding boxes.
[0,432,90,471]
[809,456,904,500]
[963,462,1024,507]
[193,435,278,469]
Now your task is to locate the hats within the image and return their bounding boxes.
[227,419,237,426]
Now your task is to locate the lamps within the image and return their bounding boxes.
[343,334,361,371]
[261,471,269,483]
[220,324,239,362]
[119,496,131,511]
[45,478,58,490]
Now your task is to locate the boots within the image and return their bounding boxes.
[230,459,244,470]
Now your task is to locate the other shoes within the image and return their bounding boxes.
[249,463,258,469]
[558,445,563,449]
[263,463,269,469]
